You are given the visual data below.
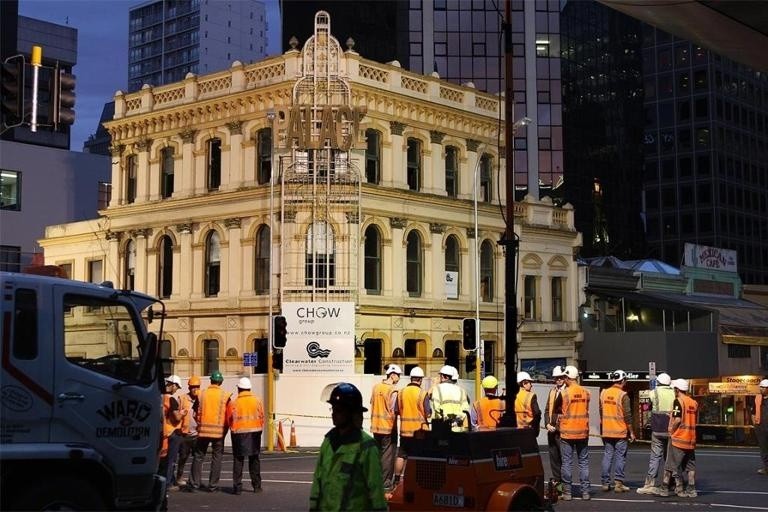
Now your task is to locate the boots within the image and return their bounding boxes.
[561,488,591,501]
[166,480,263,495]
[602,481,630,491]
[637,478,697,498]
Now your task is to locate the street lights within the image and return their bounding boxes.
[266,106,278,453]
[475,116,533,426]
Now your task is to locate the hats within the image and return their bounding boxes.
[758,378,768,387]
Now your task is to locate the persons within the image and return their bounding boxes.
[161,375,179,492]
[308,382,384,511]
[187,371,232,493]
[751,379,768,474]
[177,376,201,487]
[227,376,265,494]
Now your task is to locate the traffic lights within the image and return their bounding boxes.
[2,56,23,126]
[463,318,476,350]
[45,58,78,133]
[272,315,289,348]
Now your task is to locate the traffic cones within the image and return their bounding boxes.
[273,421,286,452]
[287,419,301,449]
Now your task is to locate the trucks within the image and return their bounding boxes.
[0,267,172,512]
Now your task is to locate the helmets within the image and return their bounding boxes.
[515,371,534,383]
[327,384,369,414]
[410,366,424,378]
[209,371,223,383]
[386,363,402,376]
[188,376,202,386]
[237,377,251,389]
[671,378,689,391]
[656,372,671,386]
[163,374,182,389]
[552,365,580,379]
[611,369,626,382]
[482,375,498,388]
[438,365,460,380]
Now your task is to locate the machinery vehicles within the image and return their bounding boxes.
[377,418,561,512]
